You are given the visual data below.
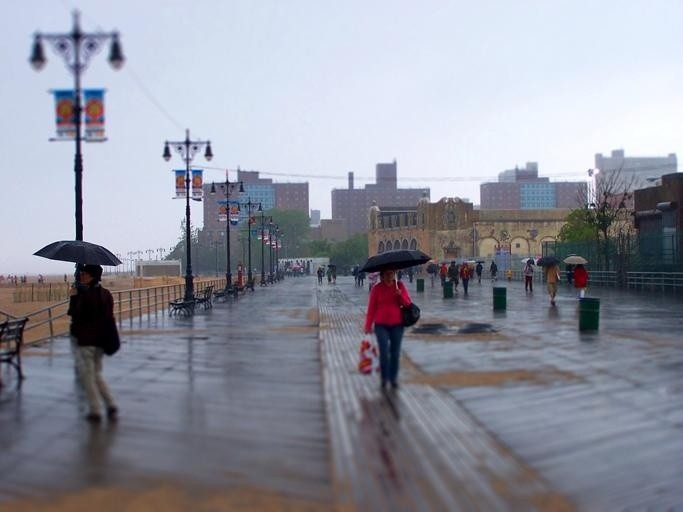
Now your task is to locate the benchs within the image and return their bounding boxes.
[167,268,284,317]
[0,315,28,381]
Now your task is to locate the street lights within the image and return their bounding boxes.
[237,215,284,286]
[100,246,173,276]
[208,168,245,288]
[236,195,262,283]
[25,7,128,288]
[645,177,661,183]
[210,238,222,279]
[161,127,213,299]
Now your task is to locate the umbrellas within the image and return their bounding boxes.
[357,249,432,277]
[32,240,123,266]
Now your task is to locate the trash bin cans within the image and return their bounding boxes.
[443,281,454,298]
[578,297,601,333]
[493,287,507,312]
[417,279,424,292]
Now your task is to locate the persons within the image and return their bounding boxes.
[524,264,535,292]
[65,263,120,423]
[366,199,380,230]
[272,268,283,283]
[490,260,497,283]
[476,260,485,264]
[352,266,377,291]
[565,264,577,289]
[0,273,67,287]
[397,263,440,279]
[439,260,483,295]
[542,264,560,306]
[317,266,336,283]
[415,190,430,229]
[286,266,304,278]
[573,264,587,299]
[362,264,412,392]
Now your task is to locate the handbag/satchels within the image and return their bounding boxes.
[360,334,381,375]
[401,303,420,326]
[101,317,120,355]
